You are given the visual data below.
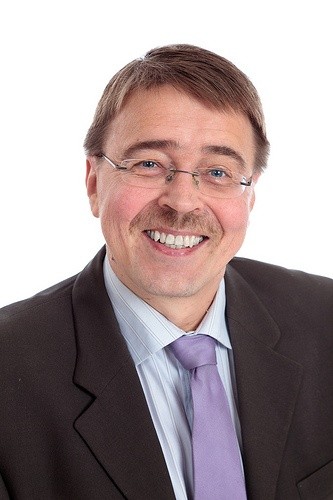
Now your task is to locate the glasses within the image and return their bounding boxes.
[101,153,255,199]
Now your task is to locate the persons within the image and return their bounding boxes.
[2,43,332,500]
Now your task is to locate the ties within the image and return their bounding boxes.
[168,334,247,500]
